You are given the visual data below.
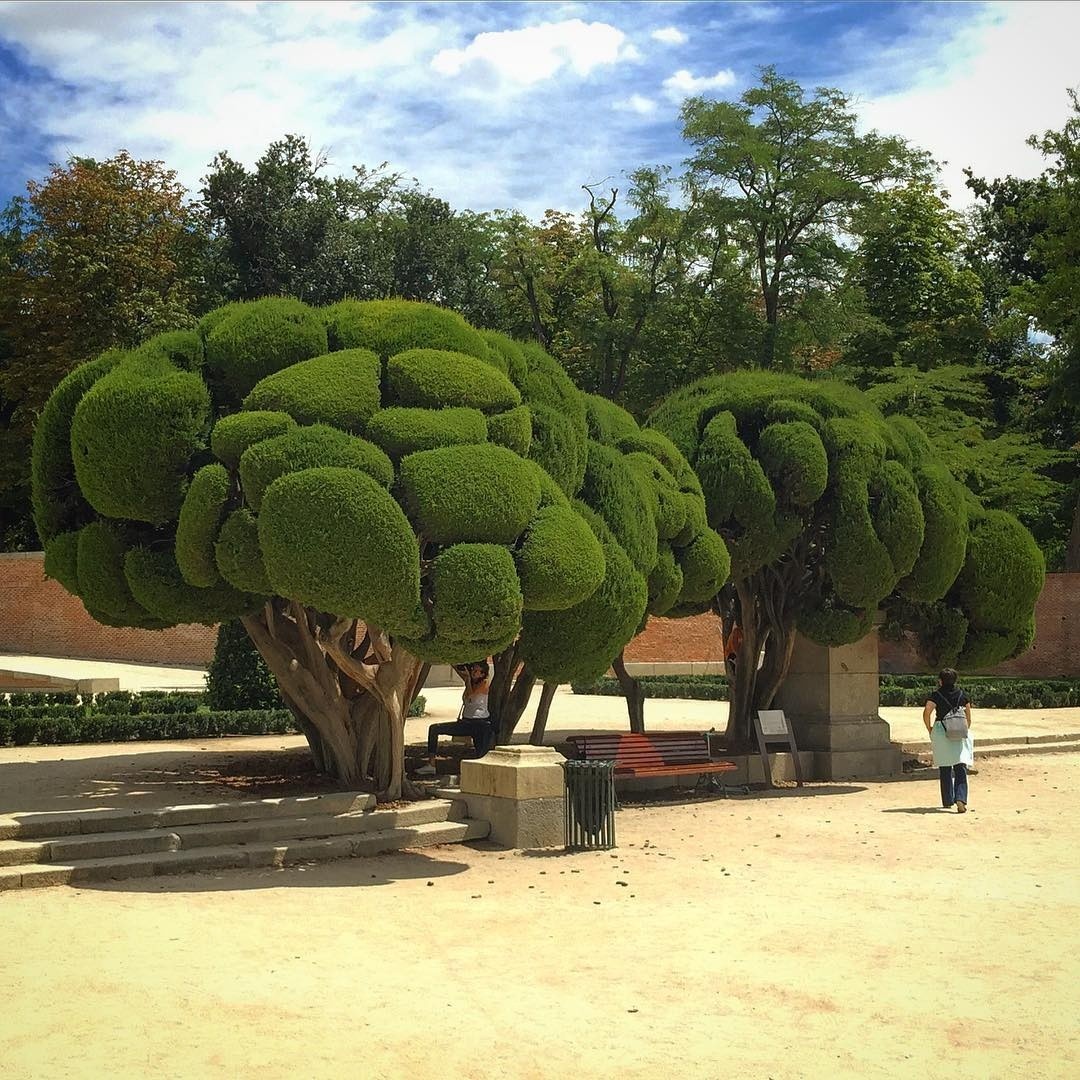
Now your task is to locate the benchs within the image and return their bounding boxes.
[564,728,737,811]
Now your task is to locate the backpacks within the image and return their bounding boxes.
[941,707,968,740]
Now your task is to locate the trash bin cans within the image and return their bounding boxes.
[554,759,617,851]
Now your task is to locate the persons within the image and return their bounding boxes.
[414,662,489,774]
[924,667,972,812]
[727,624,742,673]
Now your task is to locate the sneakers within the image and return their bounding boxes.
[956,801,967,813]
[414,766,437,775]
